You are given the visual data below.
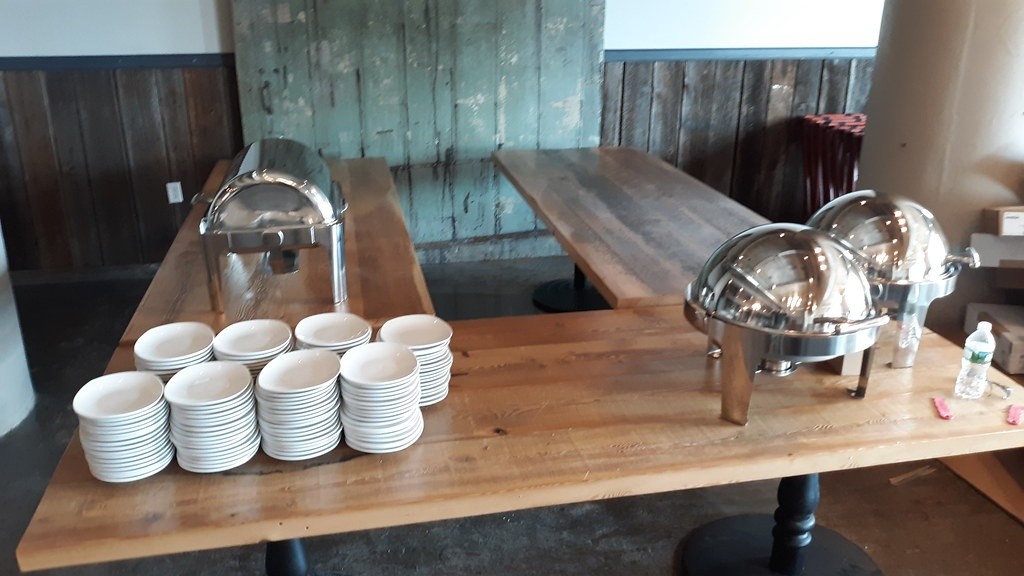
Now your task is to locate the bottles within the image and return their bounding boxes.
[955,321,996,400]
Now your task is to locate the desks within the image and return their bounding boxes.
[16,146,1024,576]
[804,111,867,220]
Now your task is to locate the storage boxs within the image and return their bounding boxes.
[963,206,1024,377]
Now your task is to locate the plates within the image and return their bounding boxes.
[72,310,454,484]
